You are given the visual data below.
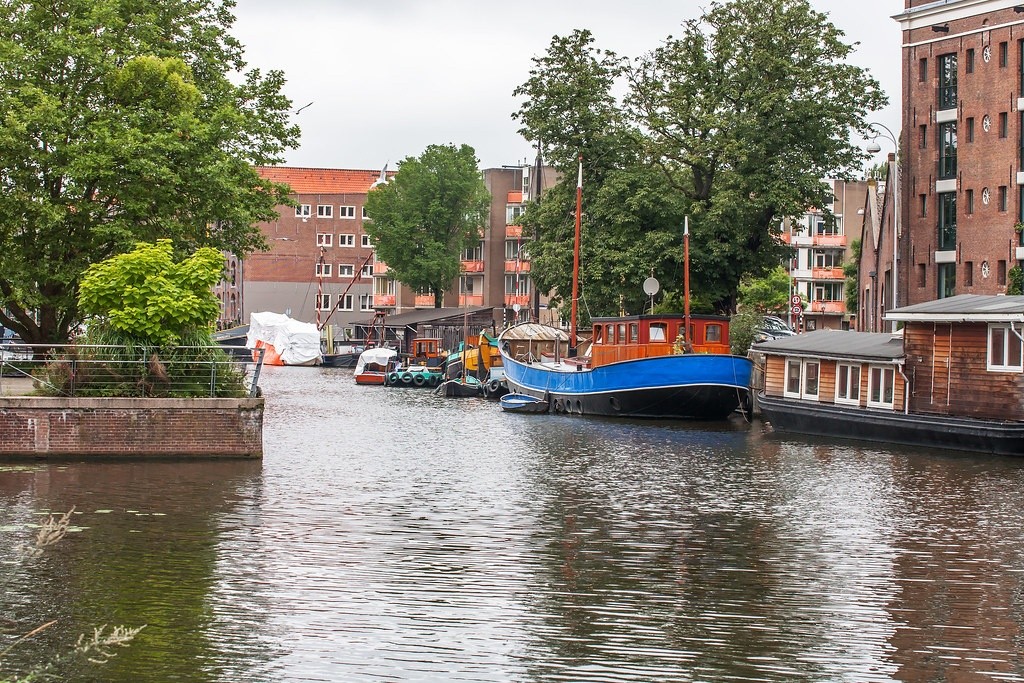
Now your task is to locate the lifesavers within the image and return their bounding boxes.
[428,375,436,385]
[413,374,425,386]
[483,385,490,398]
[389,372,399,384]
[383,374,388,386]
[489,379,500,393]
[401,371,413,383]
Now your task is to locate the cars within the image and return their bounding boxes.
[733,314,797,344]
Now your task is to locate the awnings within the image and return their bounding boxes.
[349,307,493,339]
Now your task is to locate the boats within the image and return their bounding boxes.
[745,294,1023,458]
[320,325,508,401]
[497,163,755,421]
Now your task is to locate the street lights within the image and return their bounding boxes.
[866,122,899,334]
[821,299,826,329]
